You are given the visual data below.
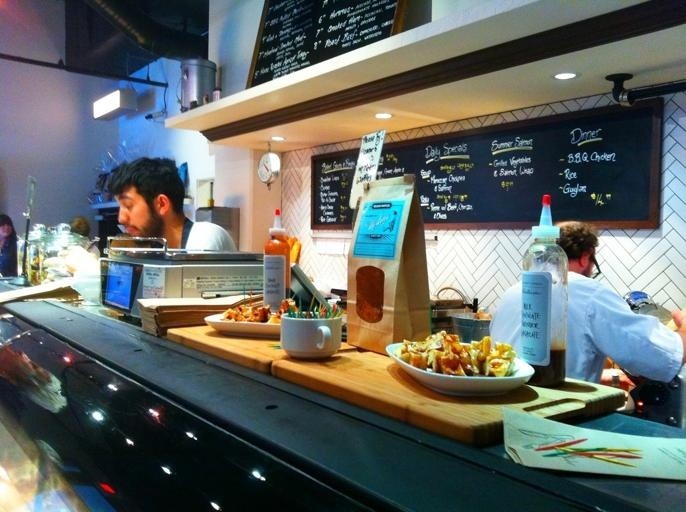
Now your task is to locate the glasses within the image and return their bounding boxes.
[589,254,602,279]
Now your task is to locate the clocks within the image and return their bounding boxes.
[258,142,279,190]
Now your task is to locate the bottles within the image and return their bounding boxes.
[262,209,292,308]
[521,194,569,389]
[610,373,620,388]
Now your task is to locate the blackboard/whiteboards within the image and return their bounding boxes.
[244,0,407,97]
[311,96,663,229]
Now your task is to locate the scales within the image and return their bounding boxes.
[100,235,336,324]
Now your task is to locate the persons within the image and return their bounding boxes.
[107,157,237,253]
[0,214,19,277]
[489,222,686,384]
[70,217,101,257]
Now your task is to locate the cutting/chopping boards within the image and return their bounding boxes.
[166,324,358,375]
[272,349,630,449]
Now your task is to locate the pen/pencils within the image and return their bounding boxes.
[534,439,642,466]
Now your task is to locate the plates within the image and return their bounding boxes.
[385,339,532,400]
[201,310,279,340]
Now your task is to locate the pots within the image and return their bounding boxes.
[179,57,217,111]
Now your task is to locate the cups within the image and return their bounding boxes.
[278,311,342,360]
